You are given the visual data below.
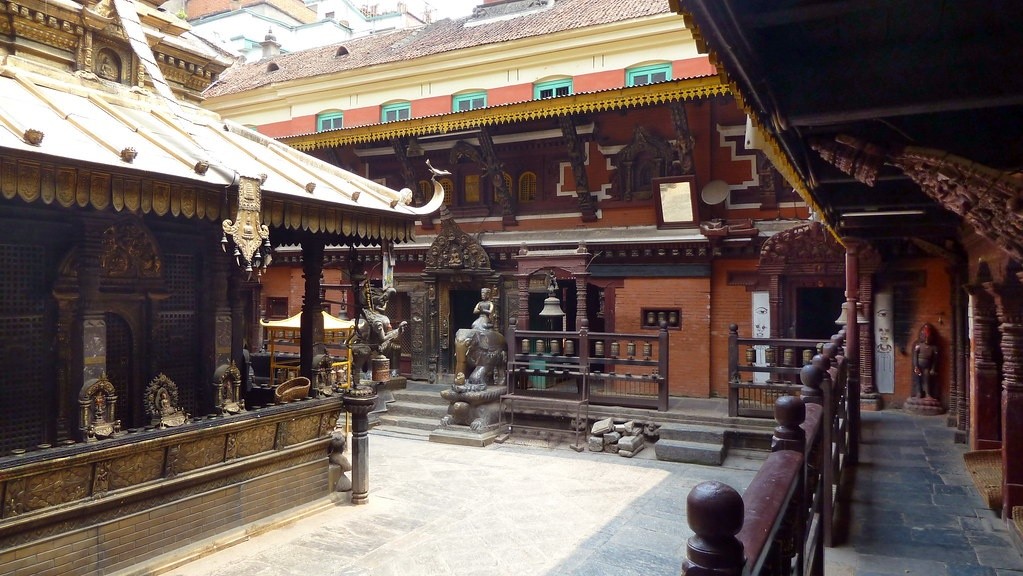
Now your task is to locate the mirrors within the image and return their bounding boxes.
[650,175,700,230]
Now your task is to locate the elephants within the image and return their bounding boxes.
[453,329,508,390]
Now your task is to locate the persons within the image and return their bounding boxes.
[913,326,938,401]
[102,57,116,77]
[161,393,175,415]
[449,248,461,264]
[471,288,494,330]
[359,285,407,352]
